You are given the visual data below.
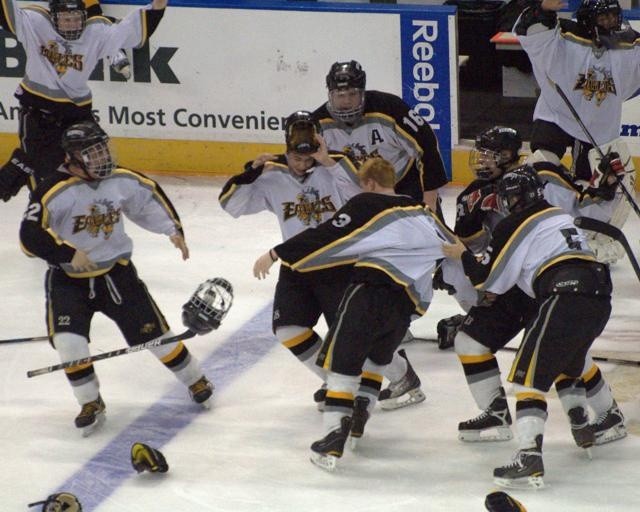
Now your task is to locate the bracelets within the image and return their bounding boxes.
[269,249,278,263]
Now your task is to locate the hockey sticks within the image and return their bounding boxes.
[572,216,640,278]
[544,71,639,209]
[25,290,216,378]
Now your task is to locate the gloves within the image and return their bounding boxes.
[589,152,625,201]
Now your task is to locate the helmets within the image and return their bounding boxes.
[57,112,112,180]
[48,1,87,40]
[576,1,623,48]
[497,164,544,216]
[324,60,366,122]
[468,125,521,182]
[286,111,322,154]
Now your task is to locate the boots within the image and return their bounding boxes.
[76,392,106,427]
[459,386,624,478]
[189,375,210,402]
[311,349,421,458]
[437,314,465,348]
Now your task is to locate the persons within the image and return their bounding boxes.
[219,111,421,403]
[16,123,213,428]
[0,0,167,196]
[254,161,453,458]
[509,0,640,227]
[441,166,613,478]
[457,125,625,436]
[309,60,456,293]
